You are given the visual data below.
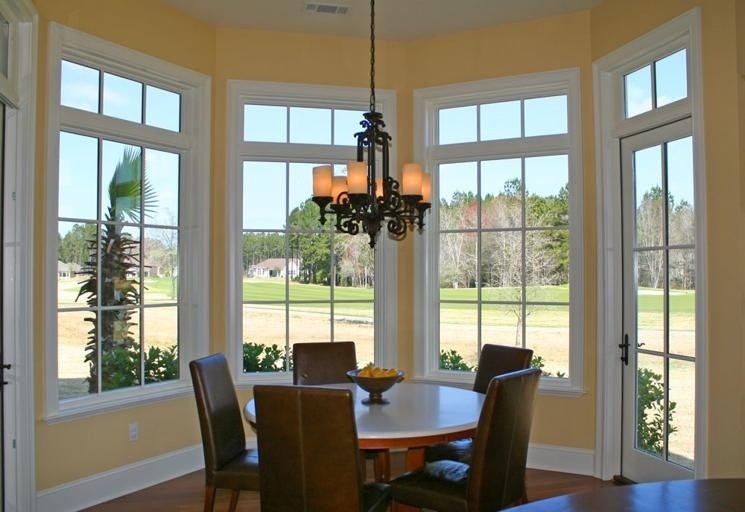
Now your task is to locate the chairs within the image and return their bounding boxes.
[292,341,389,481]
[252,383,392,512]
[189,352,260,512]
[405,343,533,474]
[387,366,544,512]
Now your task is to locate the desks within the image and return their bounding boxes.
[498,478,745,512]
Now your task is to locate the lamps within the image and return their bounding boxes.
[311,1,432,249]
